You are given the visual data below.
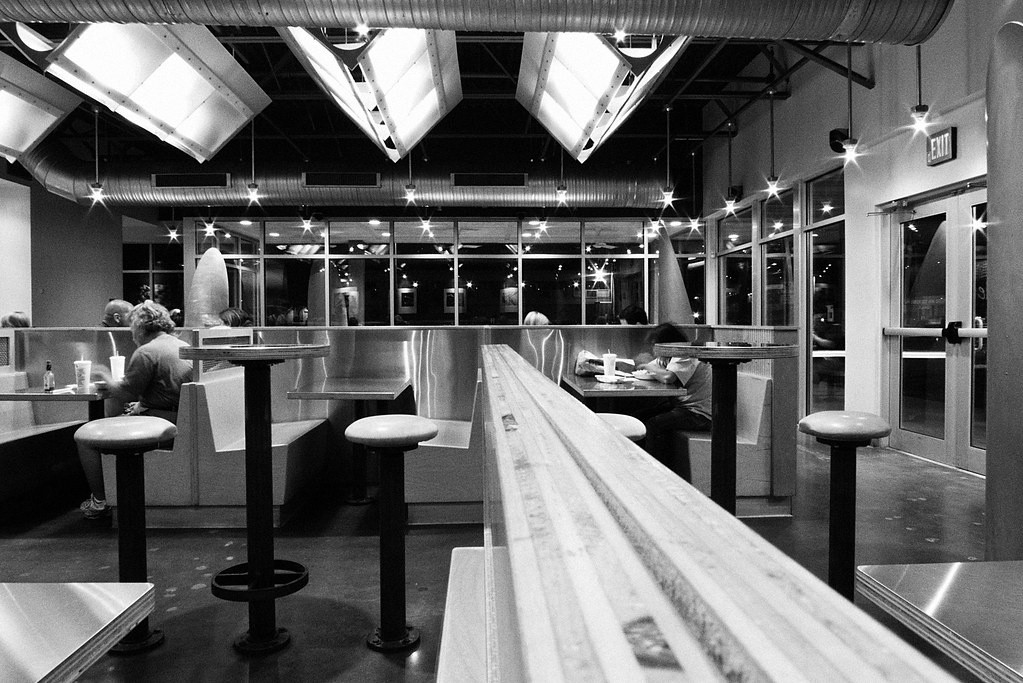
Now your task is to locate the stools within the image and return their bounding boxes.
[596,412,647,442]
[73,415,179,659]
[344,413,438,656]
[796,410,894,603]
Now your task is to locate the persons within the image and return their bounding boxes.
[211,304,255,328]
[633,322,712,459]
[63,298,197,532]
[95,297,140,328]
[812,327,845,387]
[524,311,549,325]
[0,310,43,328]
[618,304,654,325]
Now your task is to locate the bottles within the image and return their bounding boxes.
[43,360,55,393]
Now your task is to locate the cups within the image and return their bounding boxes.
[110,356,126,382]
[74,360,92,394]
[603,354,617,381]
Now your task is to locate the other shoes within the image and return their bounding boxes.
[80,493,112,519]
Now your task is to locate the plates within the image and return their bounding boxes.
[631,371,655,380]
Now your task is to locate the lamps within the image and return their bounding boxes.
[909,44,929,131]
[557,147,568,203]
[662,106,675,204]
[90,102,104,203]
[724,118,736,214]
[767,94,778,196]
[405,151,416,202]
[689,147,699,230]
[247,118,258,201]
[841,46,858,163]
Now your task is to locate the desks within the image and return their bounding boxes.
[288,377,413,506]
[854,560,1023,683]
[178,342,331,660]
[563,373,686,415]
[0,383,117,520]
[0,581,155,683]
[653,341,800,516]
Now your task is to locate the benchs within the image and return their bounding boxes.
[671,370,772,500]
[404,367,484,530]
[100,382,195,534]
[0,371,88,528]
[195,375,329,535]
